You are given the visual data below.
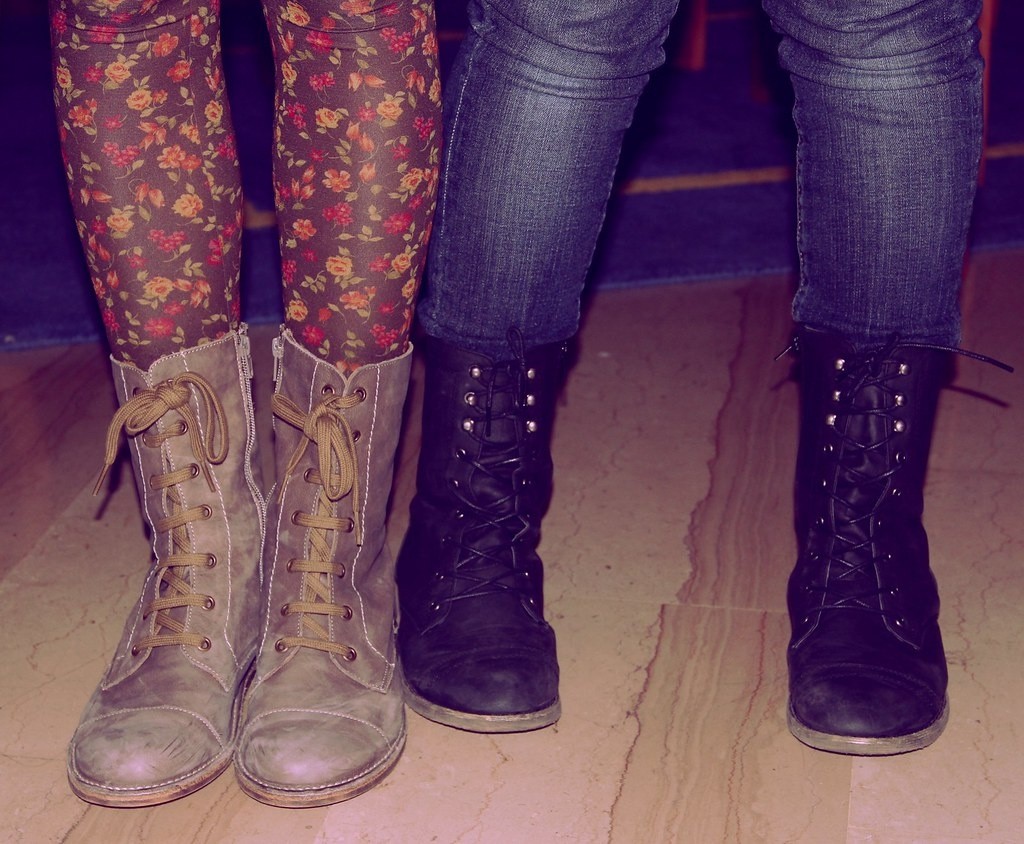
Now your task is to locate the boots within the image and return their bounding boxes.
[774,324,1013,757]
[67,321,411,809]
[395,333,583,734]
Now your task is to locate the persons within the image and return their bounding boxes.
[40,0,447,810]
[398,0,984,755]
